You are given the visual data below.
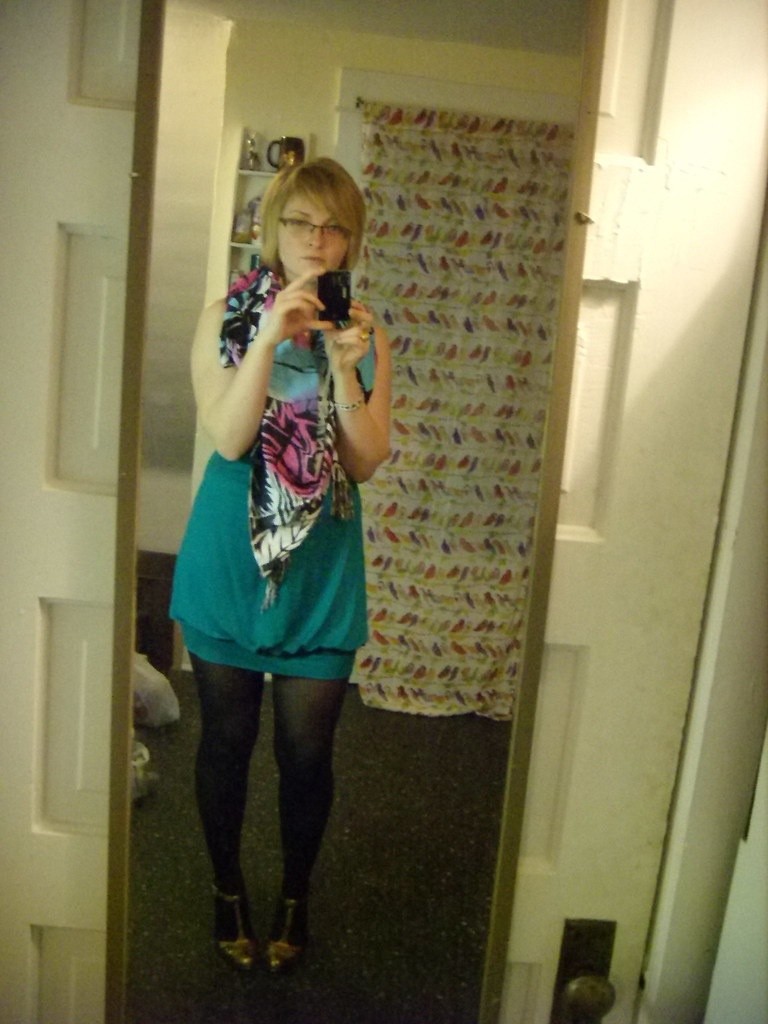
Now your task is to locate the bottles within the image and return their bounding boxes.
[230,269,240,289]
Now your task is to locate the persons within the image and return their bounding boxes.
[171,157,392,975]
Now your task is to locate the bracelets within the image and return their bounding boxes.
[334,393,365,411]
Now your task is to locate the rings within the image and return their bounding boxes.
[361,332,370,341]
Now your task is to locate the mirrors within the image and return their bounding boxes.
[105,1,609,1024]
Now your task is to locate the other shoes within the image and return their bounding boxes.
[261,895,311,973]
[212,883,259,972]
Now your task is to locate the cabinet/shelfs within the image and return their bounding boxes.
[206,126,315,305]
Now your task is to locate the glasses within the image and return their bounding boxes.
[279,217,345,239]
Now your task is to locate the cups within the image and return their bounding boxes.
[267,136,306,173]
[251,254,261,271]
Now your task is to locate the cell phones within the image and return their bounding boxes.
[316,270,351,322]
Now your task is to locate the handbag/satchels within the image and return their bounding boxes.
[132,737,159,804]
[134,655,179,728]
